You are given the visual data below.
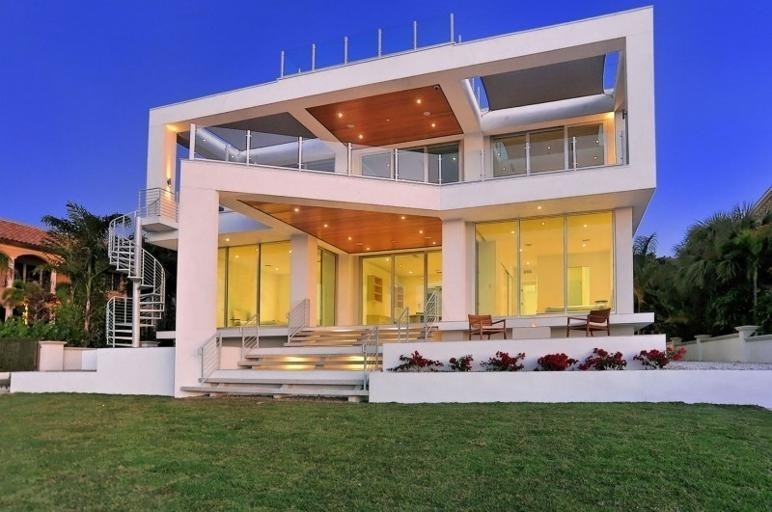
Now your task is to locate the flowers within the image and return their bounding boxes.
[577,347,629,370]
[528,351,579,369]
[449,354,476,370]
[479,349,525,370]
[385,349,443,371]
[633,346,687,370]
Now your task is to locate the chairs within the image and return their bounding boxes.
[468,314,506,340]
[568,308,611,337]
